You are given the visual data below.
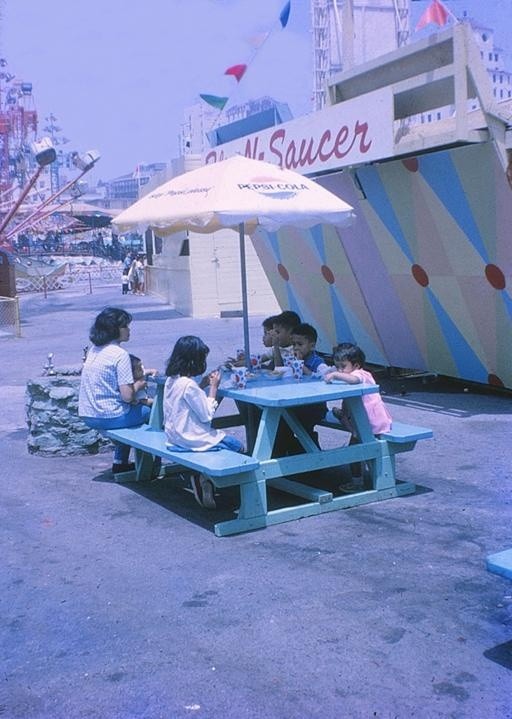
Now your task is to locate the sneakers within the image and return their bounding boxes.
[339,480,366,493]
[189,475,203,508]
[201,481,218,511]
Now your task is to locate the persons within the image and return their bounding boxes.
[127,255,141,295]
[135,253,145,296]
[268,324,329,474]
[122,270,129,294]
[163,335,247,512]
[233,310,301,370]
[128,353,159,408]
[237,314,286,367]
[323,343,392,494]
[78,308,151,474]
[123,250,133,273]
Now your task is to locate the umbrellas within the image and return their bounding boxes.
[110,155,356,365]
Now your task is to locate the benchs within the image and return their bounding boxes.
[317,408,433,489]
[100,423,261,520]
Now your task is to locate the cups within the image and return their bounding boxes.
[250,351,262,374]
[231,367,247,389]
[283,357,294,368]
[236,349,245,358]
[293,360,304,380]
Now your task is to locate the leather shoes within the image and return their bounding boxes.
[112,461,136,474]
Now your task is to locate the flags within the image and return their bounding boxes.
[132,163,139,179]
[200,93,229,110]
[414,1,450,33]
[278,1,291,28]
[248,31,268,48]
[225,64,245,80]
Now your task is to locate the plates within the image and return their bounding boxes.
[263,374,283,380]
